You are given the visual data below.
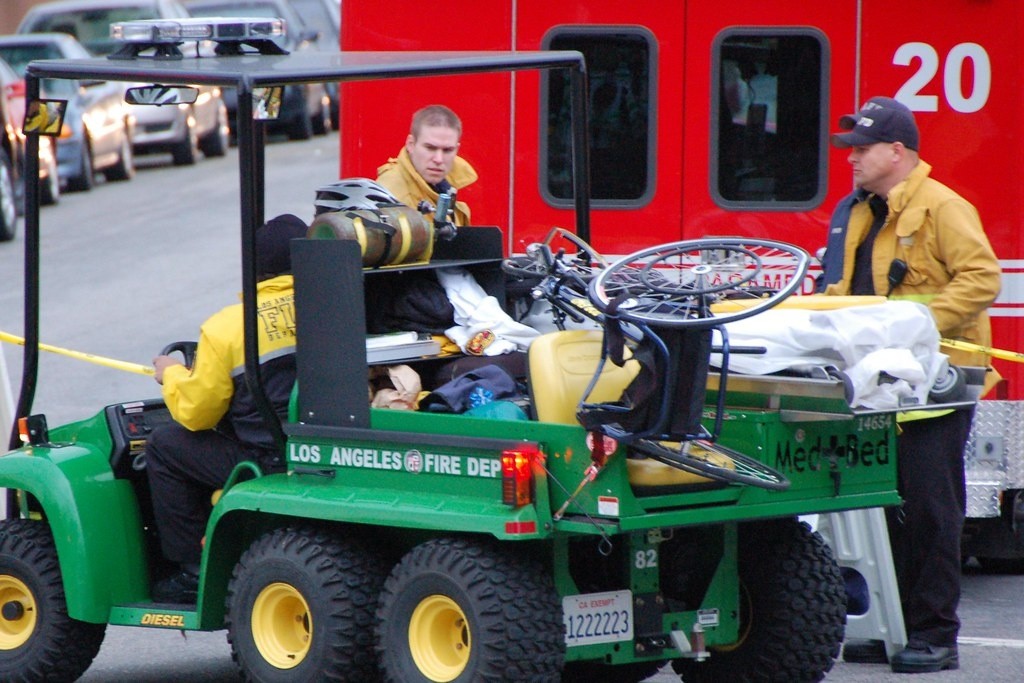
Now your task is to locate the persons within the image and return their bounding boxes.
[376,106,477,227]
[145,214,309,603]
[810,98,1004,672]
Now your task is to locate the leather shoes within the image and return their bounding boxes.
[842,633,886,664]
[890,636,960,672]
[151,565,200,602]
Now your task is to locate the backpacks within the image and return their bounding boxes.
[418,362,528,414]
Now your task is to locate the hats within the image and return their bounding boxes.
[256,214,307,275]
[839,97,912,131]
[829,109,918,153]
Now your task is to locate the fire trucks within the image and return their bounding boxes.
[335,0,1024,574]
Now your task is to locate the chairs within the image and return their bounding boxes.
[52,23,78,39]
[527,330,736,495]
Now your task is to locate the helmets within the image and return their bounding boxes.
[313,176,400,217]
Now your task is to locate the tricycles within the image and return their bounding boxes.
[501,223,810,492]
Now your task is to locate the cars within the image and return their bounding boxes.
[0,1,341,244]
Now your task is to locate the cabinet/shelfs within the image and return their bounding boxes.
[289,226,505,429]
